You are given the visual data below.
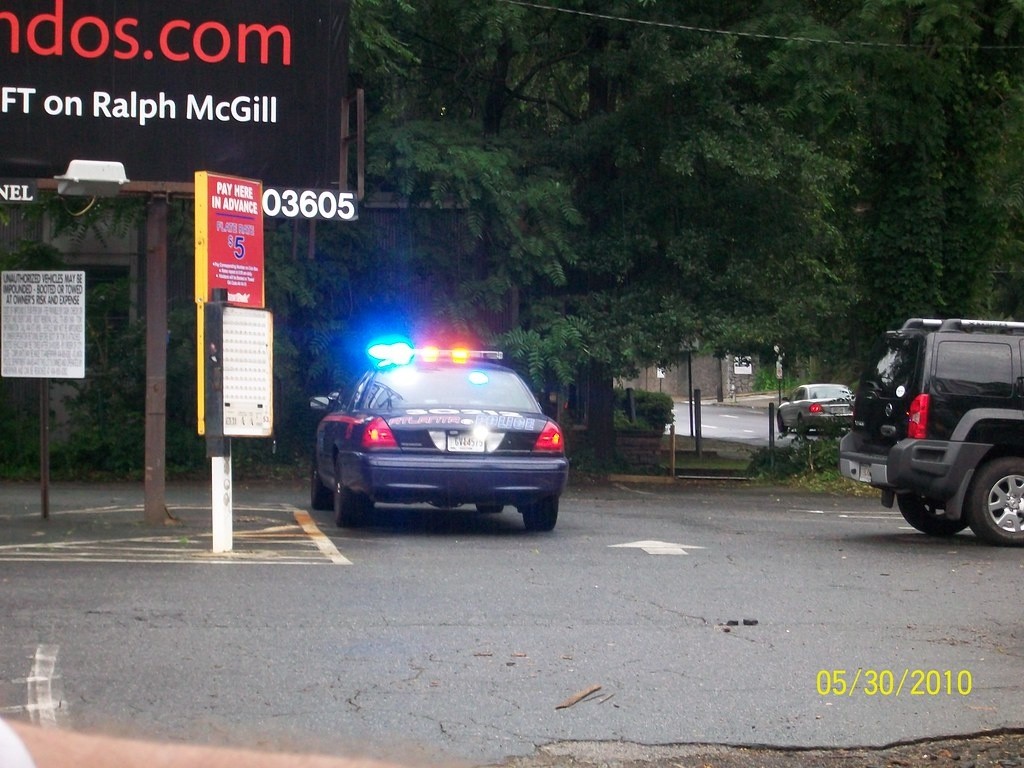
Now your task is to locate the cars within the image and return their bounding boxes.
[777,383,856,435]
[306,345,569,531]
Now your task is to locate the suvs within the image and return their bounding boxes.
[838,316,1024,545]
